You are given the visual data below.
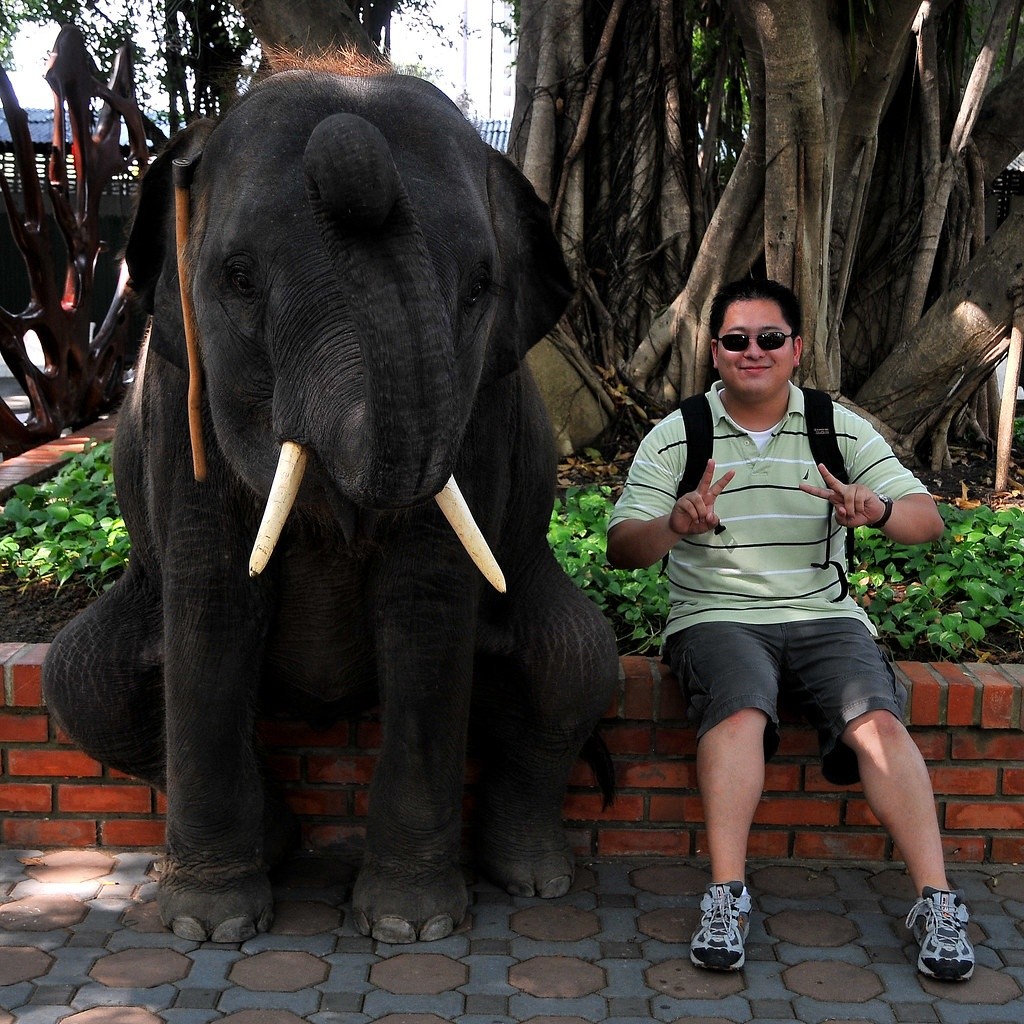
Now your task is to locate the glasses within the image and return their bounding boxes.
[718,332,791,352]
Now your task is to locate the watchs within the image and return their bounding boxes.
[865,495,893,528]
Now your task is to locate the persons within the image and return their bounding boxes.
[606,281,976,979]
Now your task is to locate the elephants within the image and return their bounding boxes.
[42,39,623,945]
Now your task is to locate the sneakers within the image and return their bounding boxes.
[690,881,753,970]
[906,886,975,982]
